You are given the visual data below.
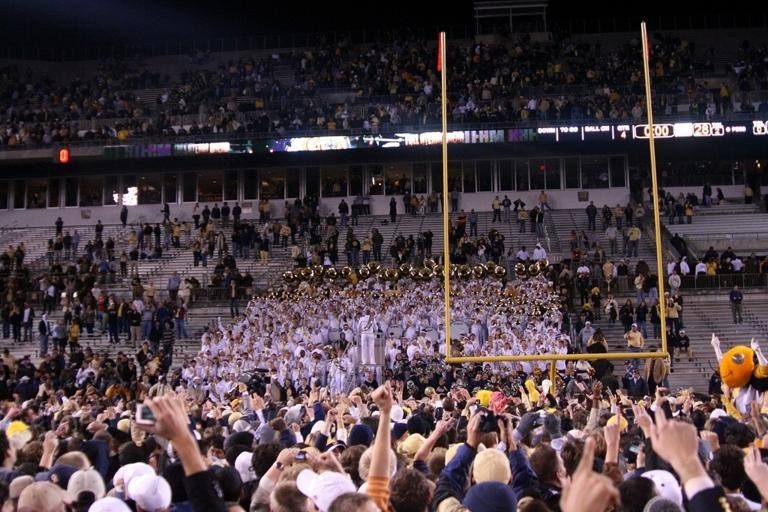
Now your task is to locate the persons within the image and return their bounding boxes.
[0,31,767,512]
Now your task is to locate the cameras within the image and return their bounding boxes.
[295,451,306,460]
[474,407,508,432]
[135,403,156,426]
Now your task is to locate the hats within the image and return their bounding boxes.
[234,451,259,483]
[8,462,173,511]
[297,466,357,511]
[638,468,684,505]
[0,346,768,451]
[470,448,512,486]
[463,482,517,511]
